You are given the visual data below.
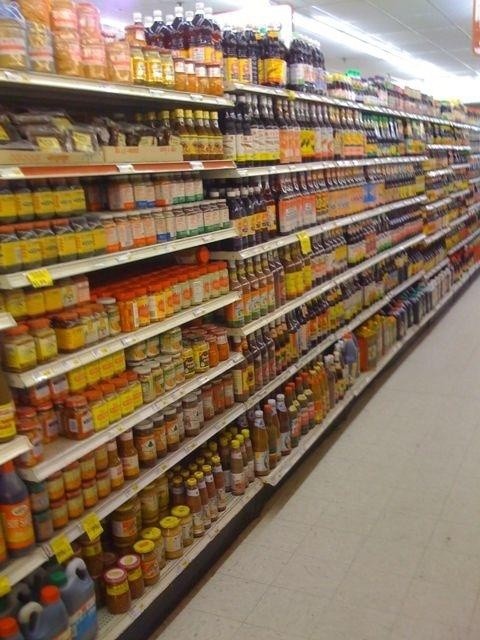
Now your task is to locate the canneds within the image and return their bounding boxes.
[0,170,233,614]
[0,0,223,96]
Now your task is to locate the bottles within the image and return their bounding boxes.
[105,428,142,490]
[225,94,479,212]
[169,400,314,546]
[129,5,223,94]
[208,171,279,250]
[356,71,480,127]
[235,213,480,402]
[1,465,37,576]
[218,20,356,101]
[132,107,225,160]
[209,258,288,328]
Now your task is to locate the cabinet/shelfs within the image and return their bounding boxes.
[1,67,479,640]
